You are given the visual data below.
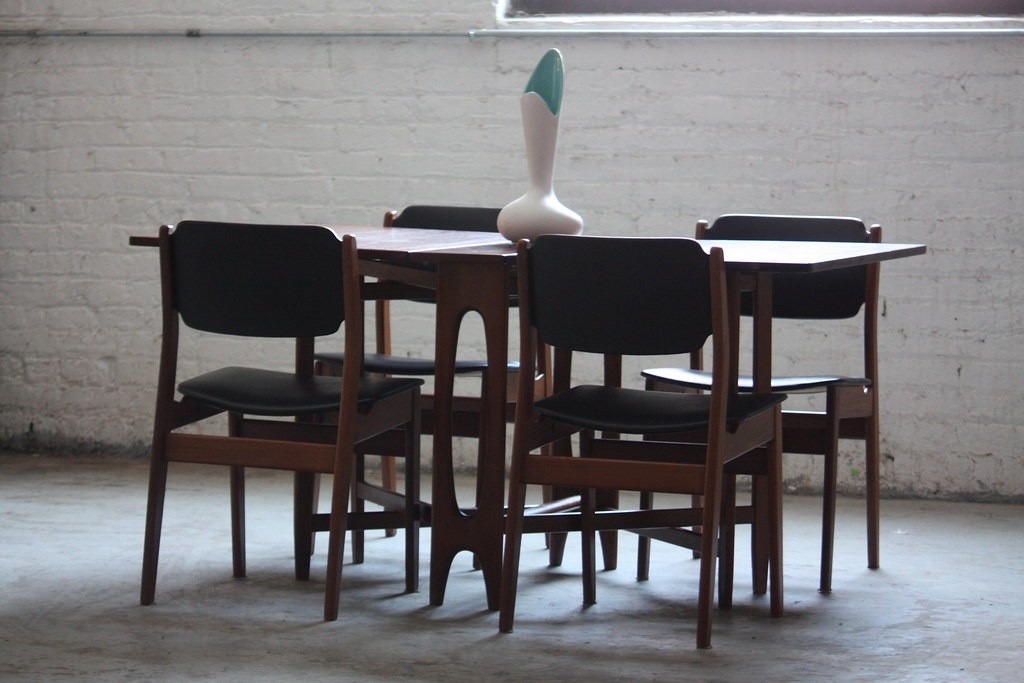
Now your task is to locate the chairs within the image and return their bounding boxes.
[691,216,881,594]
[309,205,551,569]
[139,220,425,622]
[497,235,785,645]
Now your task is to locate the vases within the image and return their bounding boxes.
[497,46,584,243]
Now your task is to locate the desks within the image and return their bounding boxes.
[129,226,928,599]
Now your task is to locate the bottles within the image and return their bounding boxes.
[496,46,586,245]
